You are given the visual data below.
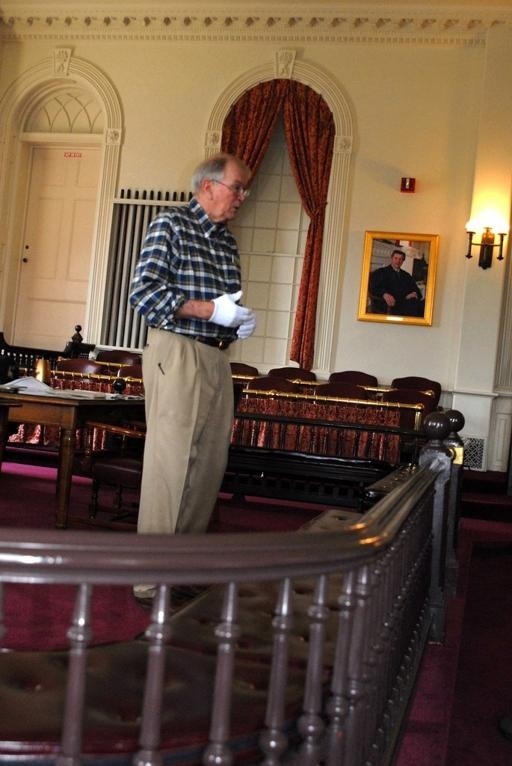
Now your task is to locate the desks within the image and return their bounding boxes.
[0,383,145,527]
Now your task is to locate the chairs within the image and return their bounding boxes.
[55,349,443,435]
[88,384,241,520]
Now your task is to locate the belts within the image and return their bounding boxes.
[150,324,229,350]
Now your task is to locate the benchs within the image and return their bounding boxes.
[0,510,362,766]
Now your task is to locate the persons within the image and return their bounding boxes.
[128,152,257,609]
[369,250,424,317]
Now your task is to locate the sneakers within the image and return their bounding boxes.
[135,582,222,615]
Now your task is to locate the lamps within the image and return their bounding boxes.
[465,204,509,271]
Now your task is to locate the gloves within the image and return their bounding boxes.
[207,290,257,339]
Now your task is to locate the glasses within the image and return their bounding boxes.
[209,179,251,199]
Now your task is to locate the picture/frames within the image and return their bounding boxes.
[357,231,441,327]
